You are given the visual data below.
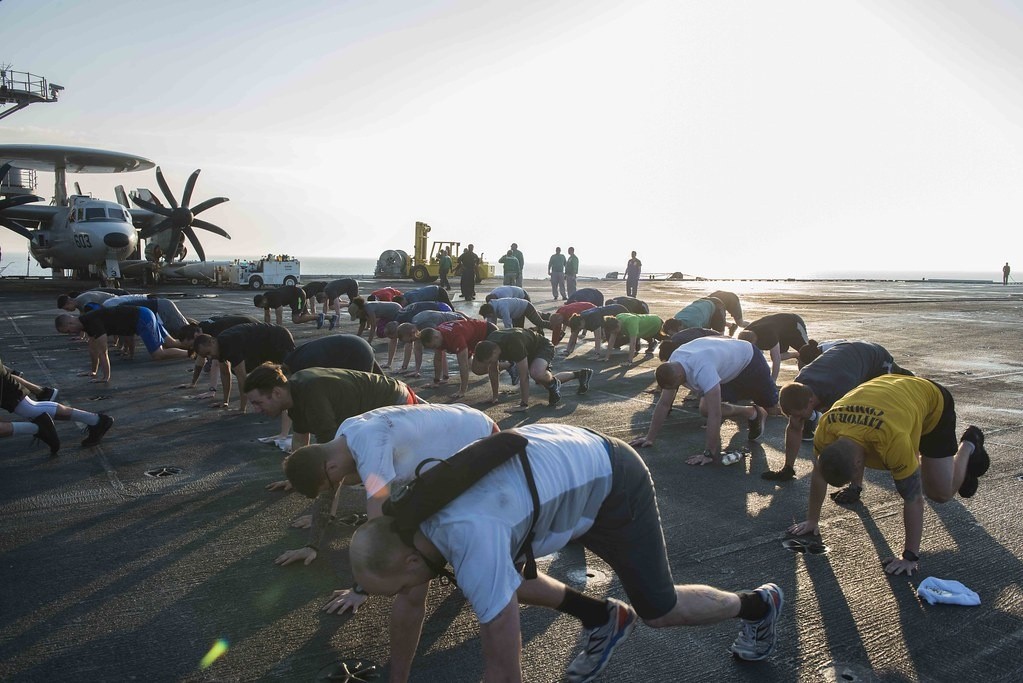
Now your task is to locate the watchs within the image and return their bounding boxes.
[902,549,920,562]
[353,583,369,596]
[209,387,216,392]
[703,449,715,459]
[848,484,862,494]
[520,401,528,407]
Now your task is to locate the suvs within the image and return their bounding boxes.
[234,259,302,287]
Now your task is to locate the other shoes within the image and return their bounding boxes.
[728,323,738,336]
[558,331,565,342]
[635,338,640,351]
[11,369,24,378]
[645,341,658,353]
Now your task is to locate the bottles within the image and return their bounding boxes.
[723,452,743,465]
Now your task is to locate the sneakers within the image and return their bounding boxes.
[549,376,561,405]
[801,409,823,440]
[317,313,325,329]
[507,361,520,385]
[959,475,978,499]
[960,425,990,478]
[748,405,768,441]
[566,597,638,683]
[578,368,593,394]
[731,582,783,661]
[28,412,60,454]
[81,414,114,445]
[36,387,58,401]
[329,315,338,330]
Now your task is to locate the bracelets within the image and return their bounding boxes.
[304,544,319,552]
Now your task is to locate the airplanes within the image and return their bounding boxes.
[0,157,233,291]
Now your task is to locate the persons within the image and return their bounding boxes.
[498,243,524,288]
[1003,263,1010,285]
[177,316,263,399]
[624,250,642,298]
[273,403,501,615]
[55,288,200,383]
[254,278,916,481]
[243,363,418,491]
[195,323,296,414]
[350,423,785,683]
[790,373,990,575]
[548,247,578,300]
[279,334,383,377]
[436,244,480,301]
[0,359,115,453]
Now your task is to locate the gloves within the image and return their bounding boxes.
[830,484,862,503]
[761,466,795,481]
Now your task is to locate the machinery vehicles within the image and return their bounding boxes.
[370,221,495,286]
